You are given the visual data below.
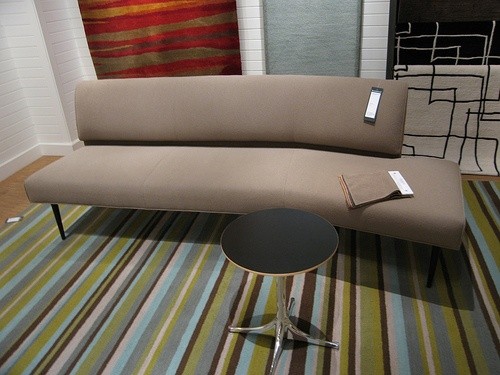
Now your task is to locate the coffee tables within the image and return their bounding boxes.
[220,208,340,374]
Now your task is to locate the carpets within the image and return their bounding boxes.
[0,181,500,375]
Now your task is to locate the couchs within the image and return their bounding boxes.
[25,75,465,288]
